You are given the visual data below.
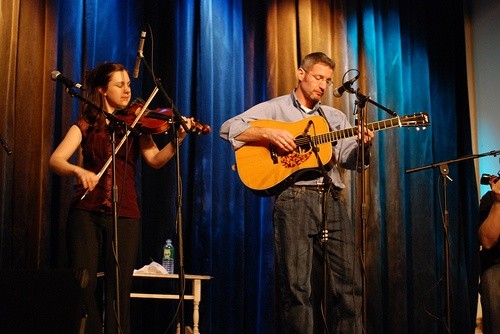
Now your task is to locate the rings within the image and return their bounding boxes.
[283,142,287,145]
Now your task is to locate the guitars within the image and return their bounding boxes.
[234,111,431,198]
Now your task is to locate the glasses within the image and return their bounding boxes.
[301,67,334,87]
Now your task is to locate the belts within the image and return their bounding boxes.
[290,183,343,193]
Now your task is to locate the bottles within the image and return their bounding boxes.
[163,239,174,274]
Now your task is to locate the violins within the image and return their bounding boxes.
[110,101,212,134]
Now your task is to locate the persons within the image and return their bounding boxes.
[219,52,374,334]
[478,178,500,334]
[48,62,191,334]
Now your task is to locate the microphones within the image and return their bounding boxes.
[51,70,87,92]
[333,75,360,97]
[301,120,313,138]
[133,24,148,79]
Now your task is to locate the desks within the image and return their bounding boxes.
[96,271,214,334]
